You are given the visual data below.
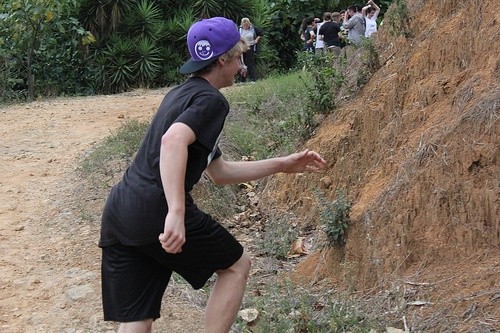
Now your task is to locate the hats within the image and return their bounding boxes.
[179,16,241,74]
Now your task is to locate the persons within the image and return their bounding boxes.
[237,18,264,84]
[300,0,380,72]
[98,16,328,333]
